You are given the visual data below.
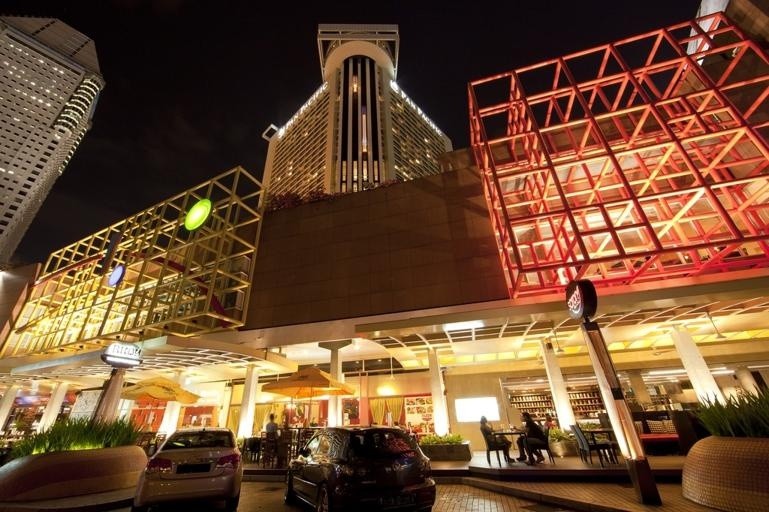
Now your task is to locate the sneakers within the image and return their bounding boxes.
[505,456,545,466]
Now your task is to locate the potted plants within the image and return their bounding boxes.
[548,427,576,456]
[680,385,769,512]
[0,414,147,512]
[419,433,473,462]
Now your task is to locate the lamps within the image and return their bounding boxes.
[553,331,566,353]
[386,357,395,382]
[709,317,728,340]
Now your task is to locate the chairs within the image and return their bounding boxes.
[569,424,620,464]
[530,426,556,465]
[241,430,315,469]
[482,433,509,466]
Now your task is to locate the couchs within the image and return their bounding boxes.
[597,409,699,456]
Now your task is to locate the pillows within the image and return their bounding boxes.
[663,420,677,434]
[634,421,644,434]
[646,420,665,433]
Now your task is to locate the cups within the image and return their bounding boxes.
[499,423,514,432]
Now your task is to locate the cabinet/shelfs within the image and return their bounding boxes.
[510,394,556,422]
[567,390,603,418]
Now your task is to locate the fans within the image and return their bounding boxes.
[646,347,675,358]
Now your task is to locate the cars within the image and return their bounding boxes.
[129,423,247,512]
[283,421,442,512]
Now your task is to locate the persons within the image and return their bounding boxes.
[265,414,279,439]
[544,414,555,430]
[513,414,526,461]
[394,421,401,430]
[480,417,515,462]
[522,412,548,466]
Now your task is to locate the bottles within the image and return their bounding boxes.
[509,391,602,418]
